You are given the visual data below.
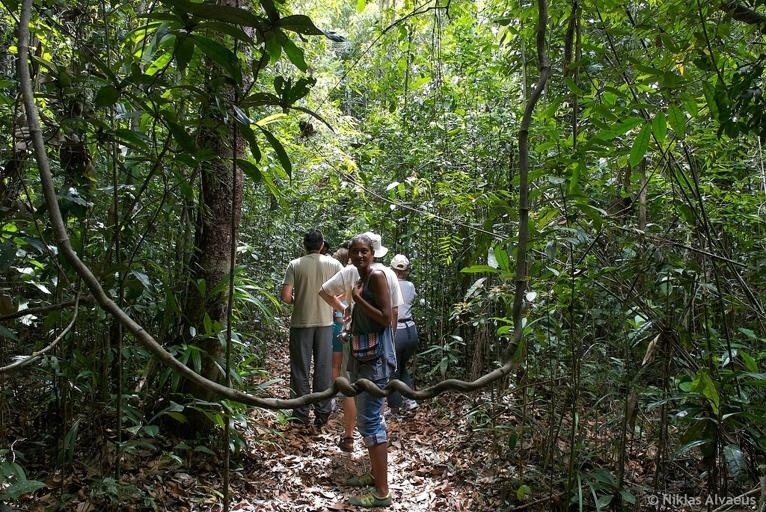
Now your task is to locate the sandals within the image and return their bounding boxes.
[333,433,356,454]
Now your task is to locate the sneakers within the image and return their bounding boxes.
[383,397,419,419]
[344,469,375,487]
[348,490,393,507]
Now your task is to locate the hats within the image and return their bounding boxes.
[363,231,390,260]
[389,253,411,271]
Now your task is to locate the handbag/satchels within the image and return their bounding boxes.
[349,332,385,362]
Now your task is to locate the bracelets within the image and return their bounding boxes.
[342,328,350,333]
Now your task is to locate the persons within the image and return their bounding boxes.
[281,230,419,453]
[336,233,396,506]
